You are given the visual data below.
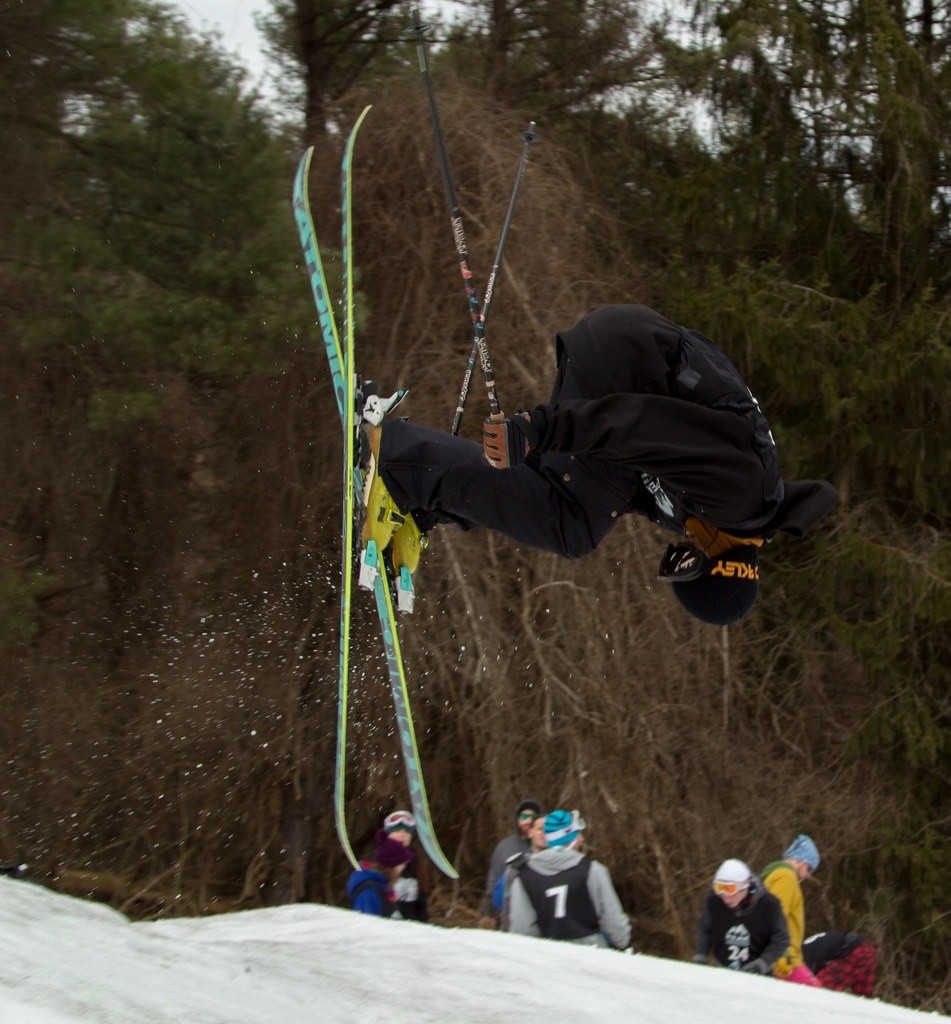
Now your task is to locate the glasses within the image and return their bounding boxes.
[657,543,707,583]
[571,809,586,831]
[713,880,751,895]
[385,812,416,828]
[519,812,538,820]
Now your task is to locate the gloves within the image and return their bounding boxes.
[743,961,761,975]
[482,408,536,472]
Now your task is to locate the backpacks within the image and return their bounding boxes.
[338,881,380,909]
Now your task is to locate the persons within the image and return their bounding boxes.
[478,801,540,929]
[692,858,790,977]
[363,304,836,626]
[801,931,877,998]
[492,816,547,931]
[759,834,823,988]
[348,837,413,920]
[376,807,431,923]
[510,810,636,954]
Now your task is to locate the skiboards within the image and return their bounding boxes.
[290,100,463,883]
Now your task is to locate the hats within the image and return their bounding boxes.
[544,811,586,848]
[378,840,414,867]
[515,800,541,817]
[384,809,415,834]
[785,835,820,871]
[715,858,752,883]
[670,543,760,625]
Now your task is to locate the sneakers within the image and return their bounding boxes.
[361,425,404,555]
[392,510,426,576]
